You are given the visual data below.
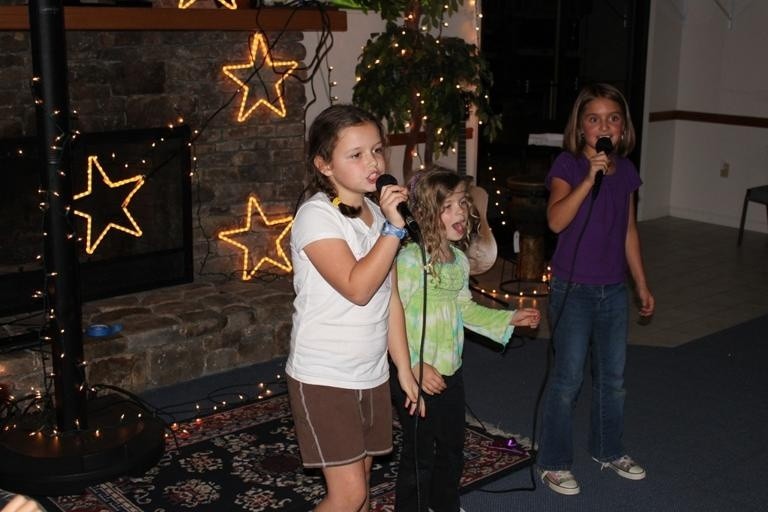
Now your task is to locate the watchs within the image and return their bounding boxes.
[379,220,406,240]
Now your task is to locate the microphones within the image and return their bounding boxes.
[589,137,614,196]
[375,173,418,231]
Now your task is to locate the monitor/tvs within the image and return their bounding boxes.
[0,125,195,320]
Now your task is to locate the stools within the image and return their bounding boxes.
[737,185,768,245]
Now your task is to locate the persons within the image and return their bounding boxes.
[536,84,655,495]
[284,105,425,512]
[388,165,540,512]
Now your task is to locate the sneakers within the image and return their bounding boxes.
[536,464,581,495]
[591,453,647,481]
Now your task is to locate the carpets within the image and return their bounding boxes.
[0,388,540,512]
